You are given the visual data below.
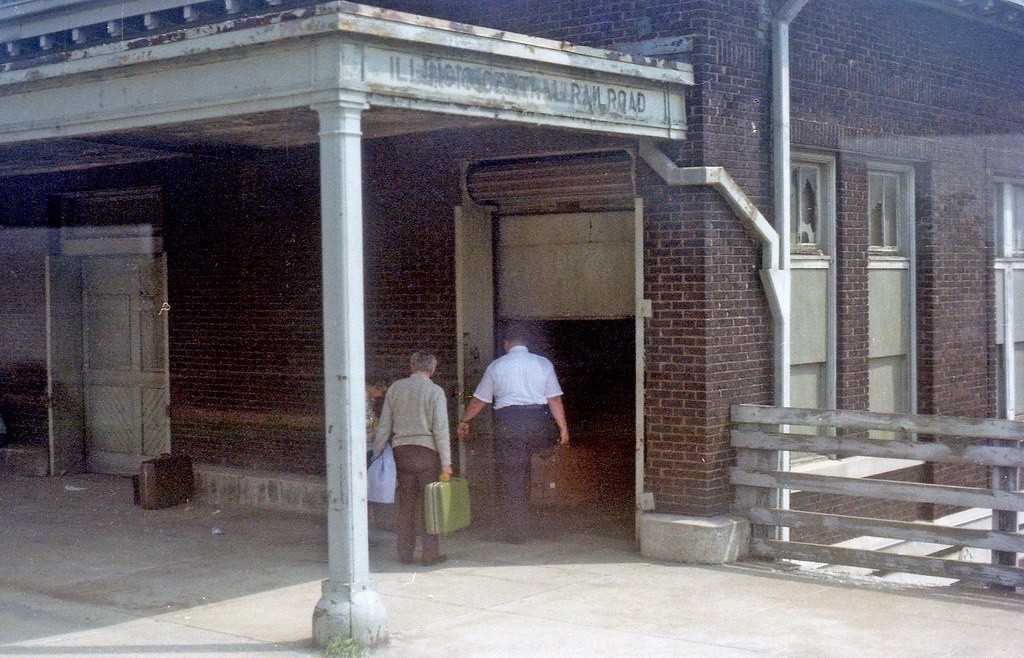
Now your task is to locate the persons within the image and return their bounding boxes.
[457,324,569,544]
[365,353,470,567]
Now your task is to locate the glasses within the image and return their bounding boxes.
[379,387,386,396]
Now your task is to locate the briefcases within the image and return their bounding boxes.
[425,477,471,535]
[139,453,194,509]
[530,445,598,507]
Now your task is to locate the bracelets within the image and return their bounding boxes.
[459,418,469,423]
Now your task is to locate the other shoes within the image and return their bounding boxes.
[423,555,447,566]
[401,560,411,564]
